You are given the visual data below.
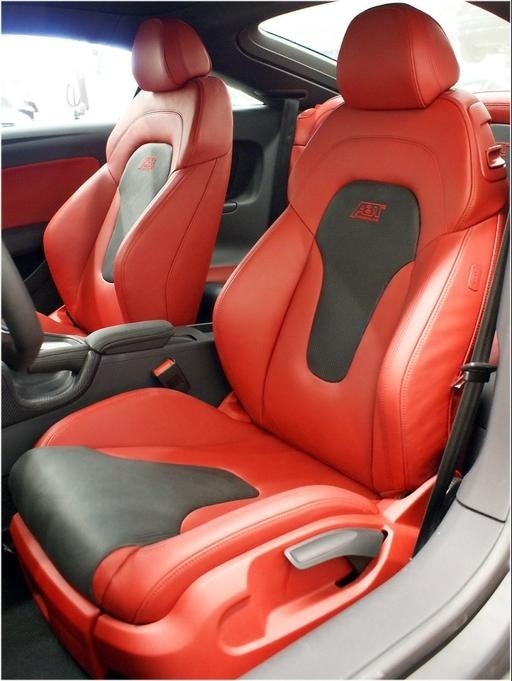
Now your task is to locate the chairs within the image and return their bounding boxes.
[2,17,234,338]
[8,1,510,679]
